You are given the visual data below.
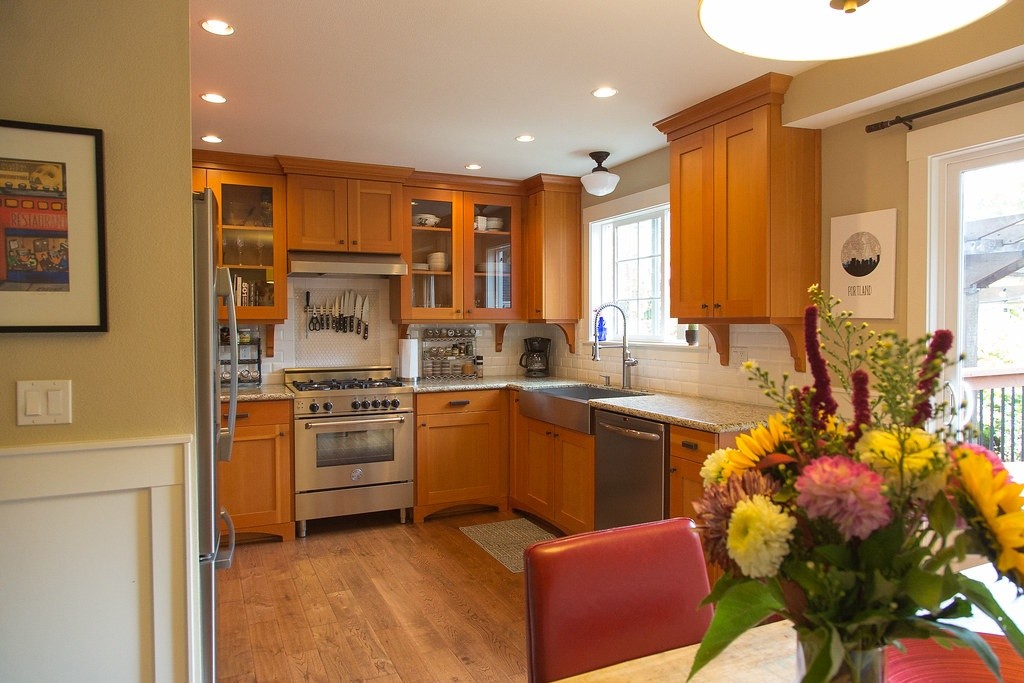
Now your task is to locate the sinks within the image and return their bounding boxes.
[535,385,650,401]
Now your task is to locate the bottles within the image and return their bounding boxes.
[598,317,607,341]
[220,324,261,343]
[424,328,484,378]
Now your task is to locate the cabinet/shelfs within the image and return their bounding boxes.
[527,174,582,353]
[286,176,402,256]
[395,179,523,324]
[669,425,772,600]
[650,72,826,375]
[411,390,511,518]
[509,398,594,536]
[211,401,293,542]
[191,167,286,354]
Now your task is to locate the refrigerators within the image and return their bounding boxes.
[190,187,238,683]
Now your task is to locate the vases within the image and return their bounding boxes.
[795,638,893,683]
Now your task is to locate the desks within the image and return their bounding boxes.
[550,562,1024,683]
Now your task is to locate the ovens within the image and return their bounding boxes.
[294,389,418,536]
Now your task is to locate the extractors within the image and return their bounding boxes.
[287,251,408,278]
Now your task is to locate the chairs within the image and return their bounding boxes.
[523,517,710,682]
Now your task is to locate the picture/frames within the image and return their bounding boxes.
[1,119,108,332]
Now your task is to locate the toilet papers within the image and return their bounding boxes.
[398,338,419,378]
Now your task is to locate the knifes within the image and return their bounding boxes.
[320,290,370,340]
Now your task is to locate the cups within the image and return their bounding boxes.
[685,329,698,345]
[412,274,435,308]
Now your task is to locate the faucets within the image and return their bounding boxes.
[592,302,638,389]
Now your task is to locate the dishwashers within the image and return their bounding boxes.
[594,410,669,538]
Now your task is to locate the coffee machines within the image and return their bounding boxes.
[519,337,552,377]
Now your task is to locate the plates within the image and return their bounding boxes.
[412,262,429,270]
[477,261,510,272]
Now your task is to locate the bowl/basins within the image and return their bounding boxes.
[428,252,449,271]
[474,215,503,231]
[412,213,441,227]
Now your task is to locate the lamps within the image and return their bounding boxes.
[700,0,1002,61]
[582,150,620,198]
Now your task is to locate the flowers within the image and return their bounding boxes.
[678,285,1024,683]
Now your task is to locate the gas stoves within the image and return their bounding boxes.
[285,365,414,391]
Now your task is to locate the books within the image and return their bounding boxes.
[233,274,274,306]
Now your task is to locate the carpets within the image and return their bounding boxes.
[458,517,556,576]
